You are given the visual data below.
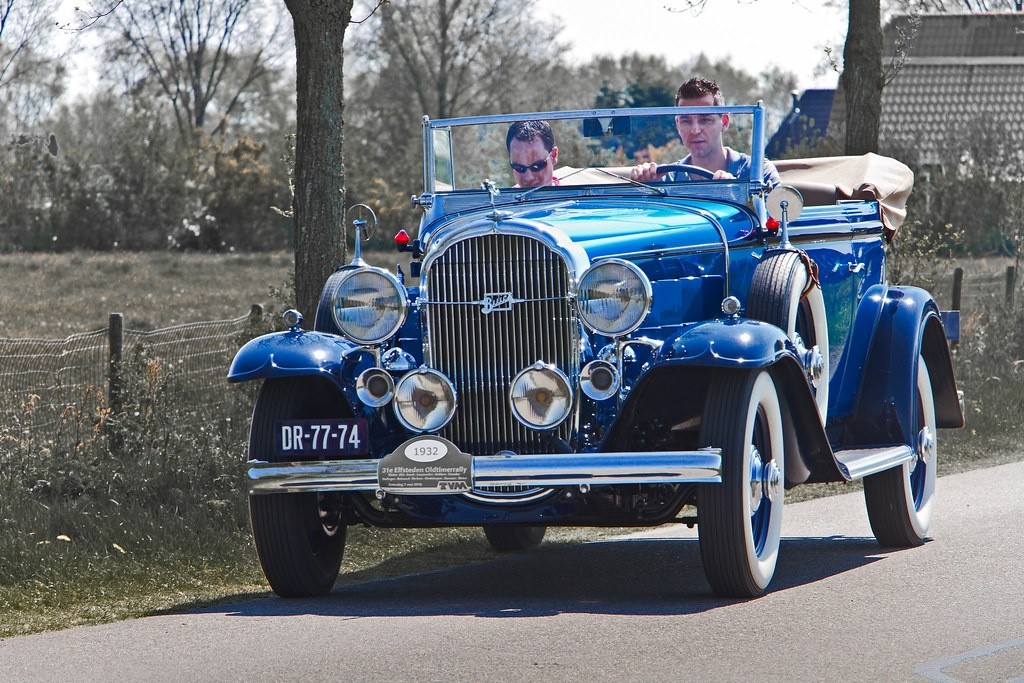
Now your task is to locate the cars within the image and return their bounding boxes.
[223,99,968,600]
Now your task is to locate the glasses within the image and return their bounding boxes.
[508,151,555,174]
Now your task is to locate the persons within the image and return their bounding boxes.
[629,77,781,198]
[506,120,563,188]
